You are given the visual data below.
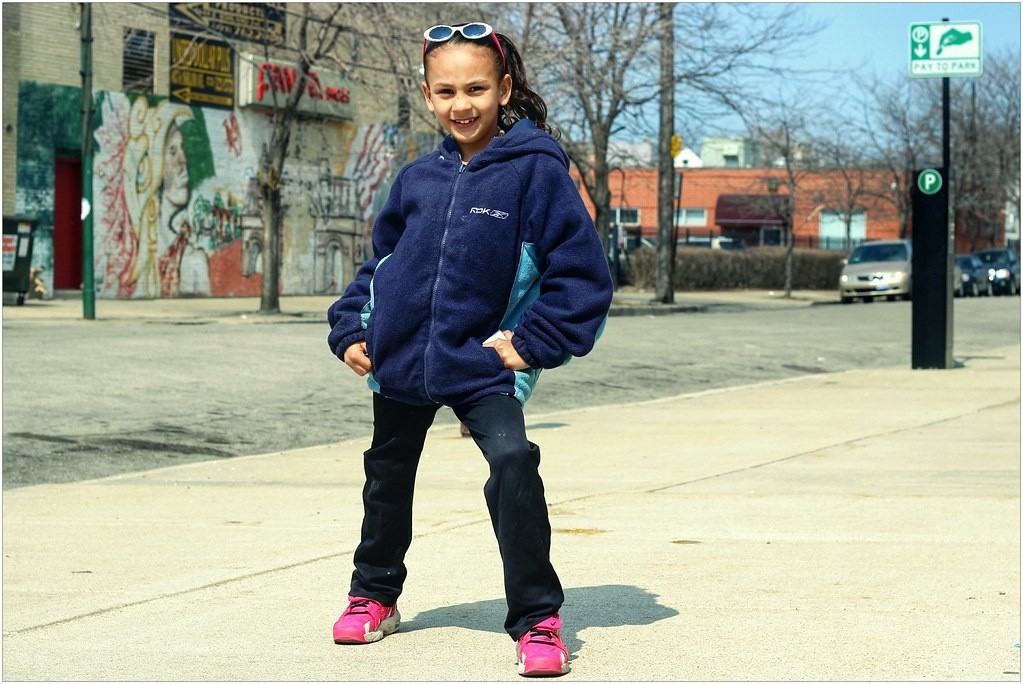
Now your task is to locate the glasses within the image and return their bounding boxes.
[422,21,504,70]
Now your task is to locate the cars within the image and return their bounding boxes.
[608,235,655,260]
[972,249,1021,297]
[676,236,736,250]
[955,255,993,297]
[838,239,912,304]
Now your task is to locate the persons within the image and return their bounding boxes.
[326,22,616,676]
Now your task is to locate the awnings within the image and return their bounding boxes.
[712,194,790,229]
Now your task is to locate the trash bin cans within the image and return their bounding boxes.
[2,213,42,306]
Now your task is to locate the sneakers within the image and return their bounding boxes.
[333,596,401,644]
[516,612,570,677]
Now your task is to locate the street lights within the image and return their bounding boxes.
[768,177,794,298]
